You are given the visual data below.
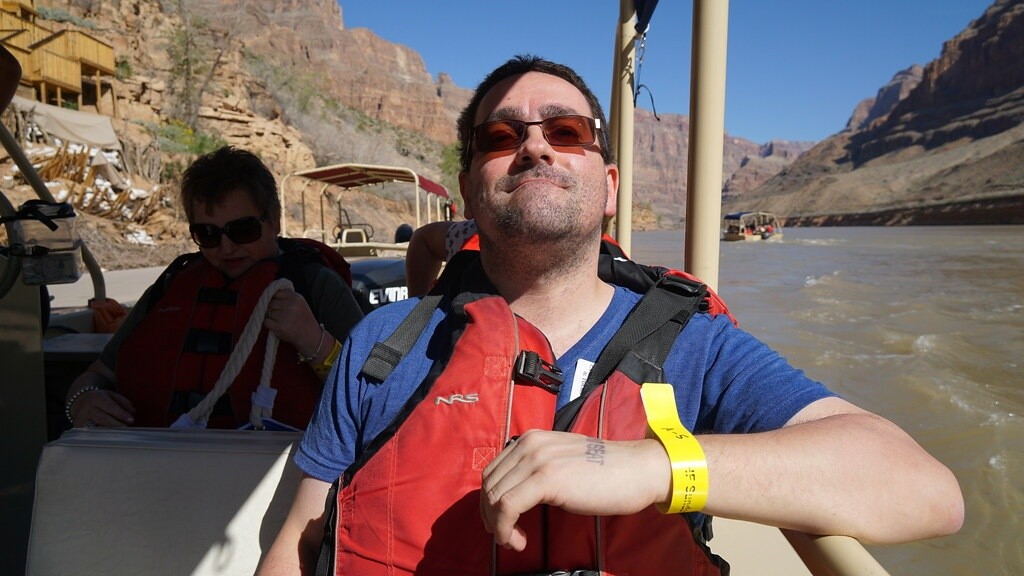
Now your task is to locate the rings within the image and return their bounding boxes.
[266,311,274,317]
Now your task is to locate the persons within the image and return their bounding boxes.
[65,145,366,431]
[394,224,414,244]
[728,224,773,233]
[255,54,965,576]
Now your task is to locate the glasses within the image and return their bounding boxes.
[189,206,271,249]
[465,115,612,169]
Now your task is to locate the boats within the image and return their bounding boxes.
[722,211,783,242]
[279,163,457,281]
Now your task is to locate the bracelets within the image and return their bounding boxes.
[65,385,101,423]
[640,382,707,515]
[313,340,344,375]
[297,323,326,365]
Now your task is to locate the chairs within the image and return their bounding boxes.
[406,221,478,298]
[341,228,367,243]
